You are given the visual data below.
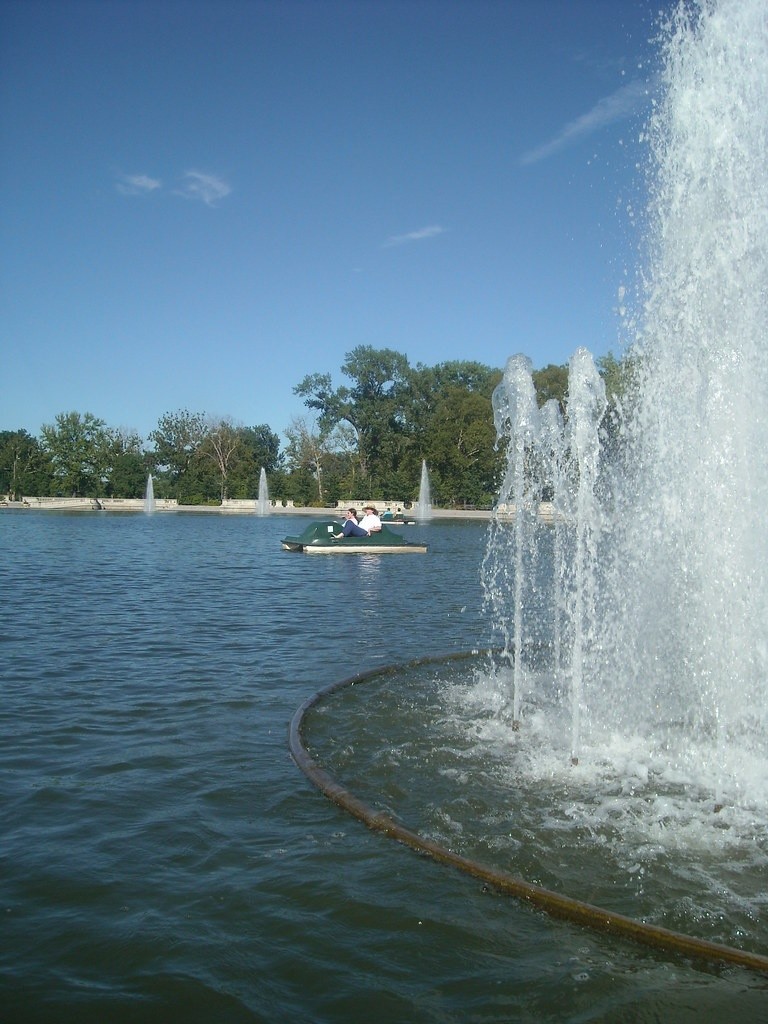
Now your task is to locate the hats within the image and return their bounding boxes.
[362,504,378,515]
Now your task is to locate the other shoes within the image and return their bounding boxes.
[330,533,337,538]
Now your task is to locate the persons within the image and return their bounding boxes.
[341,508,359,526]
[394,507,403,517]
[331,503,382,538]
[383,507,393,518]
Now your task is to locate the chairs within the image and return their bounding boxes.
[385,514,393,518]
[90,499,106,510]
[395,514,403,518]
